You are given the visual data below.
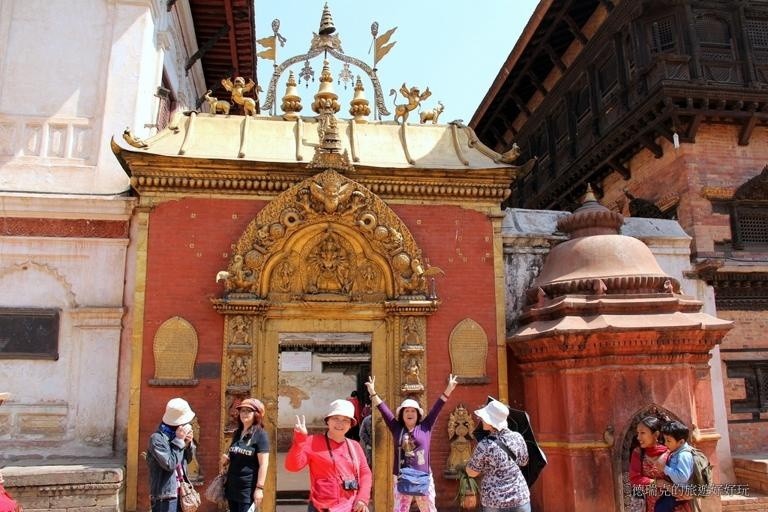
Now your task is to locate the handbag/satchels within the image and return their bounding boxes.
[178,480,203,512]
[206,471,229,504]
[396,464,431,497]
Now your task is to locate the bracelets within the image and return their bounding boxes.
[255,486,263,491]
[368,393,377,397]
[442,392,449,400]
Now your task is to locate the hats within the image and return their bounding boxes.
[472,397,510,432]
[394,397,425,426]
[323,396,359,429]
[235,397,267,417]
[160,397,195,426]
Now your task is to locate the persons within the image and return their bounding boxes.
[220,398,270,512]
[364,373,458,512]
[344,391,361,443]
[628,416,693,512]
[361,264,377,292]
[359,413,372,471]
[280,260,291,289]
[146,396,196,512]
[284,398,372,512]
[340,191,366,220]
[361,394,372,418]
[654,419,696,512]
[464,400,531,512]
[297,188,319,221]
[308,246,349,295]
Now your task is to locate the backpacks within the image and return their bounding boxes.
[665,448,716,499]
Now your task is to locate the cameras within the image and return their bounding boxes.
[344,480,358,490]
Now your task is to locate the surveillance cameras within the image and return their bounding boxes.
[673,133,679,148]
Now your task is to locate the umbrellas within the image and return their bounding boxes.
[470,395,547,490]
